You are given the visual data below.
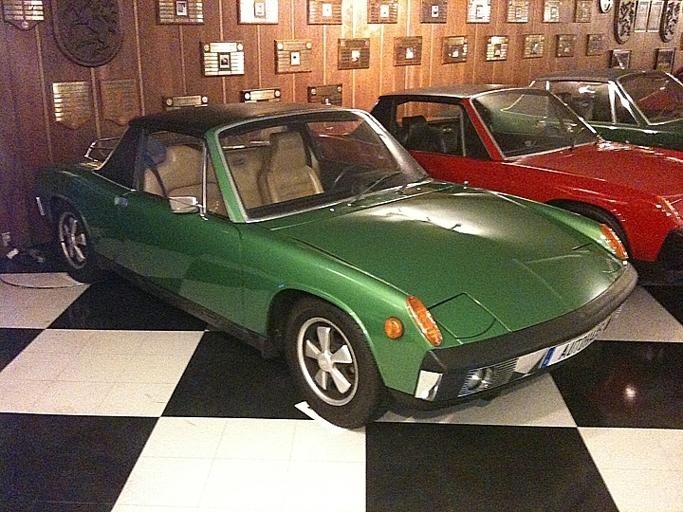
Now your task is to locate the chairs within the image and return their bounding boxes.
[400,110,495,160]
[594,87,619,124]
[144,131,325,216]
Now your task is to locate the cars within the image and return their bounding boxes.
[314,84,682,286]
[36,102,640,431]
[479,68,682,156]
